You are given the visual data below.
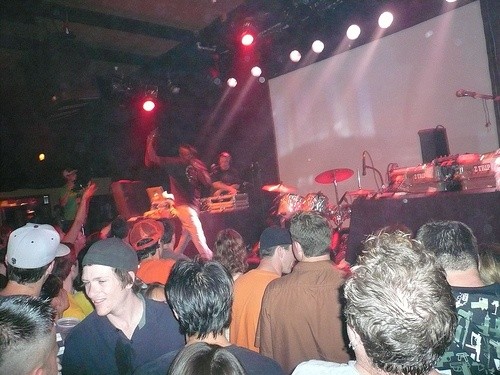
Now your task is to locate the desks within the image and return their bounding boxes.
[345,192,500,265]
[174,212,257,260]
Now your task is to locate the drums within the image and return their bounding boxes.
[306,193,330,216]
[278,194,305,218]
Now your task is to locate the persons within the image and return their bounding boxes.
[56,166,80,232]
[145,130,213,261]
[210,151,242,203]
[0,182,500,375]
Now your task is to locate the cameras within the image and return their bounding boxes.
[146,123,161,144]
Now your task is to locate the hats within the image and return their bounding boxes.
[129,218,165,251]
[259,225,292,248]
[82,237,139,273]
[6,222,71,269]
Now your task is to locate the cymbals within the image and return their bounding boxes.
[315,168,354,184]
[347,189,374,195]
[263,184,296,192]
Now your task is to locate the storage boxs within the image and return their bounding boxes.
[208,193,250,212]
[405,163,497,192]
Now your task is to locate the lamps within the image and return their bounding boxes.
[108,12,394,112]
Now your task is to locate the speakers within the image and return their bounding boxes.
[345,189,500,263]
[419,128,450,164]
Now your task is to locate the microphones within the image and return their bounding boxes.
[363,152,366,175]
[456,89,495,101]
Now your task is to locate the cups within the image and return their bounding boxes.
[56,317,80,344]
